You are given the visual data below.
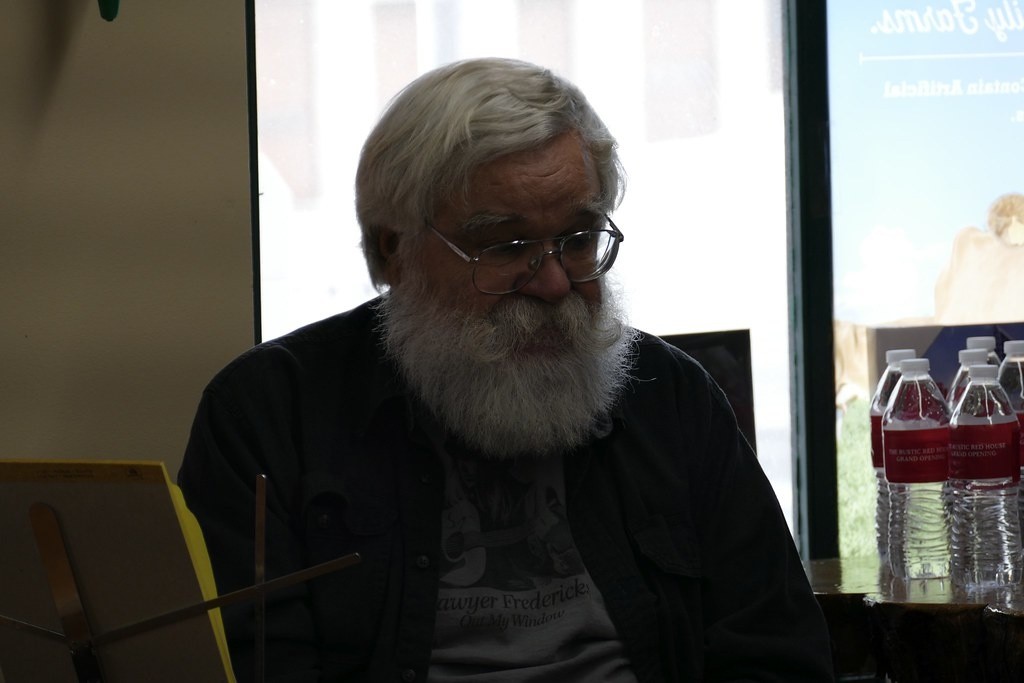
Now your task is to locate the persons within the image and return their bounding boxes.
[178,62,832,683]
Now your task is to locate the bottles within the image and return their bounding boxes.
[880,357,952,579]
[944,336,1024,593]
[868,348,918,566]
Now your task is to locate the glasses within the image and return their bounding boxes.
[425,212,624,295]
